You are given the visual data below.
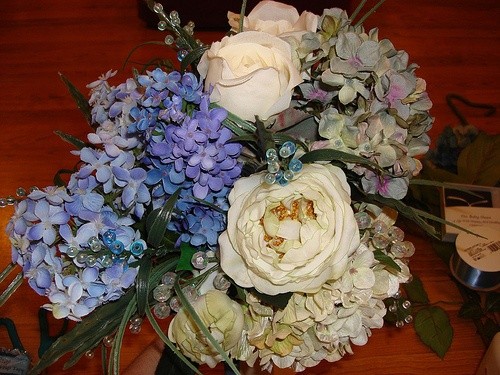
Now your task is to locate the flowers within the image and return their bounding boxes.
[0,1,488,375]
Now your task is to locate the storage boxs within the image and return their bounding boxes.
[439,183,499,243]
